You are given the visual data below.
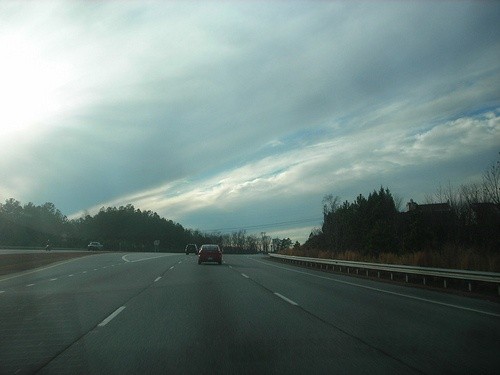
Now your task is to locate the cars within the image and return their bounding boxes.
[196,245,222,265]
[88,241,104,251]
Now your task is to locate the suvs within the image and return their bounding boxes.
[184,243,198,255]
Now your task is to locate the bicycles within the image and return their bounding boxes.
[44,244,51,252]
